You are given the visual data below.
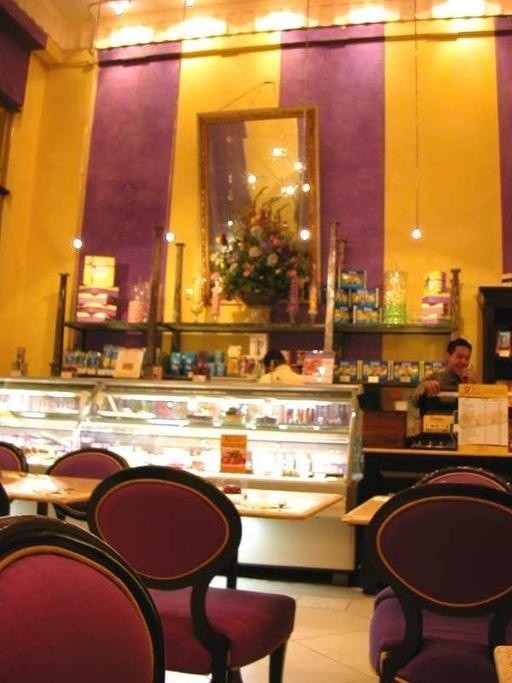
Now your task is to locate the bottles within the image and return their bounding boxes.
[277,403,351,427]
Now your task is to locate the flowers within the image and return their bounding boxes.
[209,184,312,302]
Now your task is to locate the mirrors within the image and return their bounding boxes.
[196,105,320,308]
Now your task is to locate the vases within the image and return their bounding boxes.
[239,292,274,307]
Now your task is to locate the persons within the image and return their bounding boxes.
[257,349,316,383]
[411,338,471,406]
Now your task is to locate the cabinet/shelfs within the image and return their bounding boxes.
[48,226,461,388]
